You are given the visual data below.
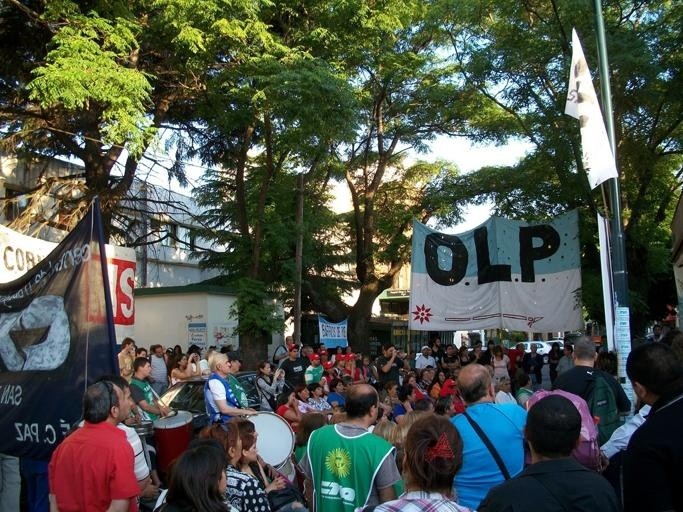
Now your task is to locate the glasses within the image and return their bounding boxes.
[103,381,113,414]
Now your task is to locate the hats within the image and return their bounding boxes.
[289,343,299,350]
[309,349,357,369]
[227,351,240,360]
[421,345,429,353]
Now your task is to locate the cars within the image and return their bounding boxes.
[545,340,566,351]
[509,340,555,365]
[159,371,296,416]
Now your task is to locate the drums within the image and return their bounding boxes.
[129,421,154,437]
[244,411,295,472]
[154,410,194,471]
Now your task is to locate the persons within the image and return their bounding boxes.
[473,393,623,510]
[296,381,403,511]
[2,323,683,511]
[447,361,530,511]
[152,445,238,511]
[374,408,475,511]
[46,375,144,511]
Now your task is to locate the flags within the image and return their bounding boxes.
[562,27,618,192]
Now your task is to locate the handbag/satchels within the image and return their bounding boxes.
[525,388,602,470]
[587,376,620,447]
